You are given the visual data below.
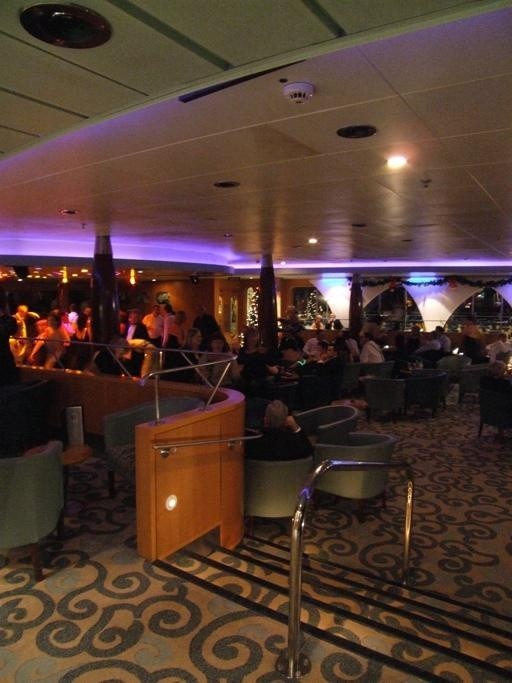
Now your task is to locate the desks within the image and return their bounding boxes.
[25,443,93,505]
[381,368,448,409]
[265,371,299,414]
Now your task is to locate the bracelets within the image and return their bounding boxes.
[293,426,302,434]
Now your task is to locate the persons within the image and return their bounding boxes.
[260,400,314,460]
[1,302,240,386]
[238,307,385,399]
[389,316,511,396]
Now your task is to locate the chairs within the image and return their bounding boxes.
[362,360,395,378]
[246,456,313,536]
[459,362,490,392]
[336,363,362,399]
[367,378,407,425]
[296,404,358,441]
[312,431,394,512]
[437,355,471,387]
[0,441,63,582]
[464,336,486,362]
[103,397,204,499]
[479,375,512,439]
